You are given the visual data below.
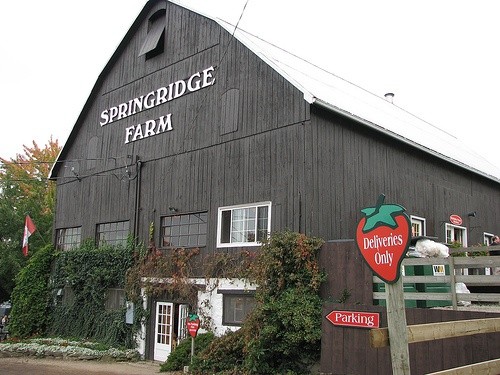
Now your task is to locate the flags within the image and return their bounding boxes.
[22,214,36,257]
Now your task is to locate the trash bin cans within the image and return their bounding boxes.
[373,250,416,309]
[409,236,458,308]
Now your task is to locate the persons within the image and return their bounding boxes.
[489,235,499,275]
